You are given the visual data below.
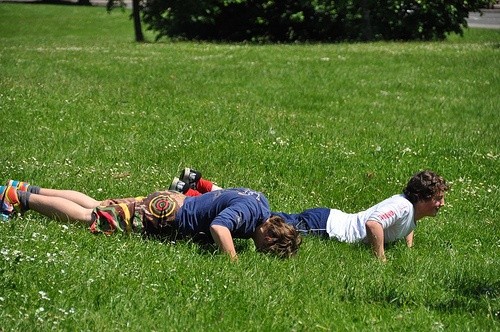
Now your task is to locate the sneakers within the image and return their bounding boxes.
[169,177,189,193]
[6,180,30,191]
[0,185,22,225]
[179,167,202,190]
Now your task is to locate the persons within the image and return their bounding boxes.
[2,175,304,266]
[168,167,451,262]
